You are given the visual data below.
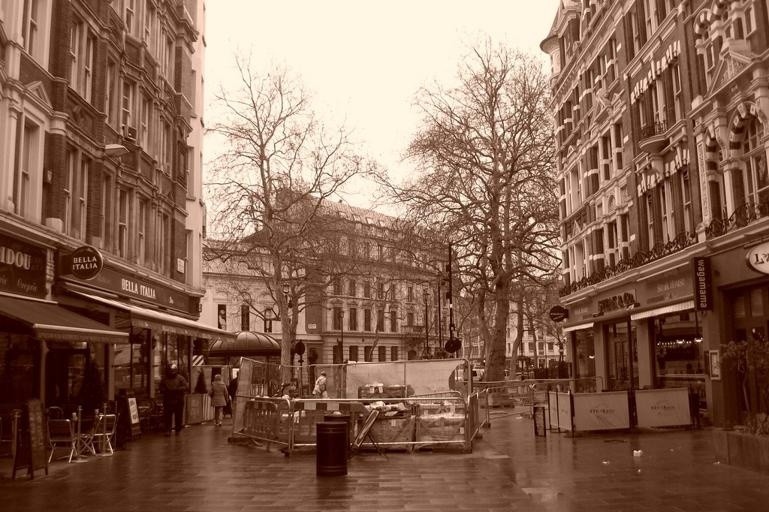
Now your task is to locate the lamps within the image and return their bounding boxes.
[105,144,130,157]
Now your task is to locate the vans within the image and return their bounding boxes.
[504,369,510,380]
[471,368,485,381]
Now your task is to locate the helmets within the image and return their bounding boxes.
[166,364,177,377]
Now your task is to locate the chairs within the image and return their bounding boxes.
[47,407,117,463]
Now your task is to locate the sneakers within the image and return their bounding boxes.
[312,385,321,395]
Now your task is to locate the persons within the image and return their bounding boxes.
[160,364,189,437]
[312,371,326,396]
[75,360,103,453]
[208,374,228,426]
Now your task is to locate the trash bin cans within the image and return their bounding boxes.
[323,416,350,459]
[316,421,348,477]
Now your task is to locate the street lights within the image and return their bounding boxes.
[421,288,433,359]
[283,280,293,309]
[338,309,344,364]
